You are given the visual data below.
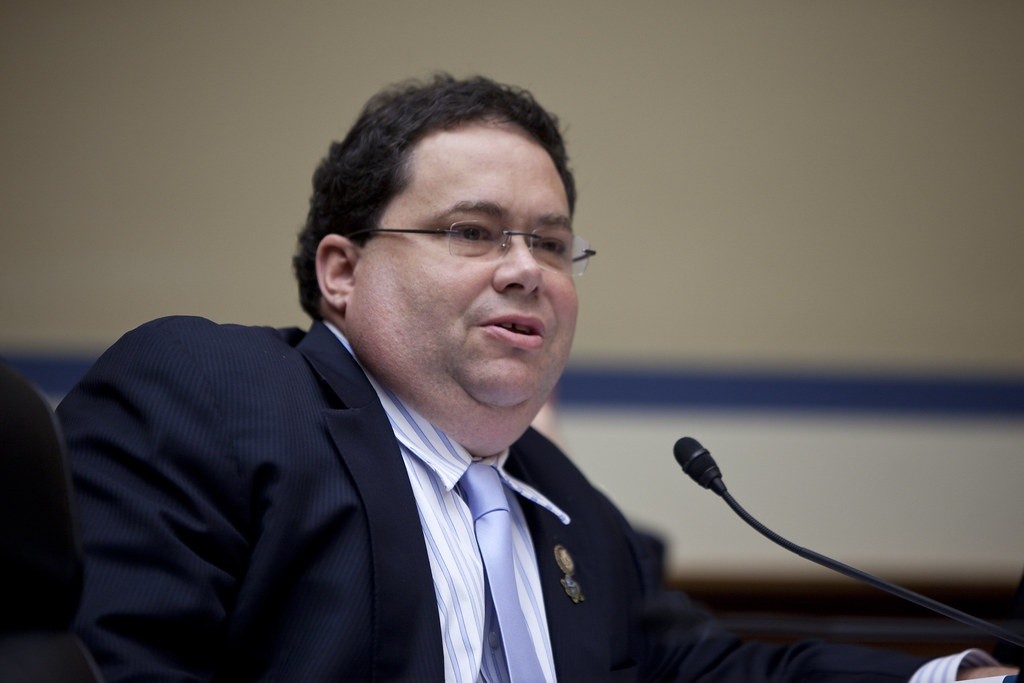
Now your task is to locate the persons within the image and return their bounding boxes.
[53,71,1024,683]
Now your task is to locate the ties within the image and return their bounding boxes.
[458,464,544,682]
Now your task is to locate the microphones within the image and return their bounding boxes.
[673,437,1024,648]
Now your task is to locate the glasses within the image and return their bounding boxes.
[345,220,596,276]
